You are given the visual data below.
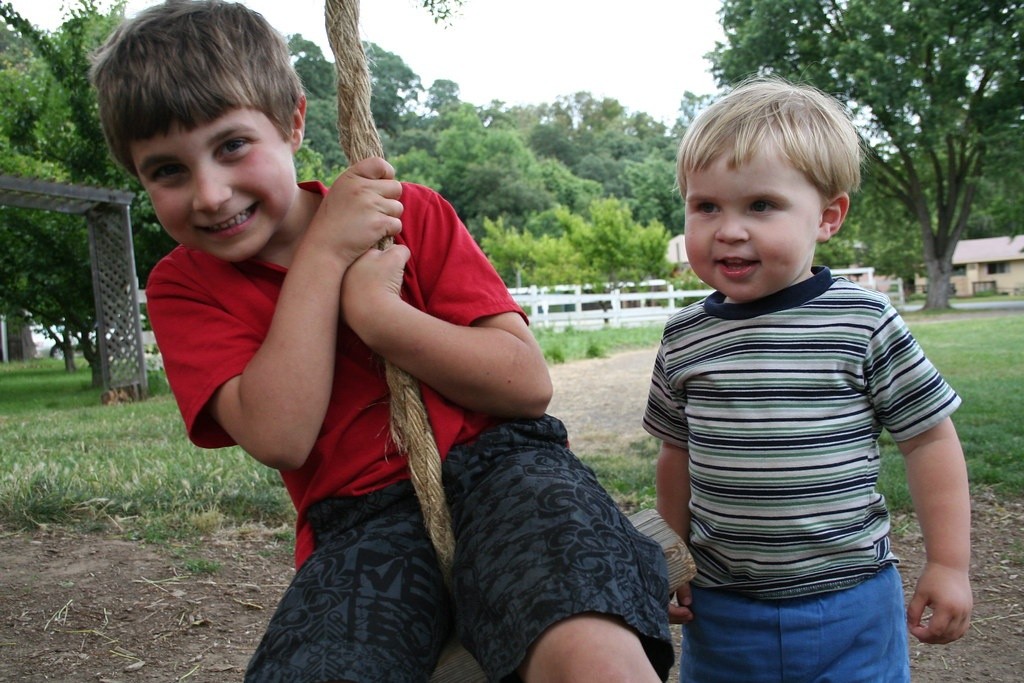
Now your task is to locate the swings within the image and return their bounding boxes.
[326,0,698,682]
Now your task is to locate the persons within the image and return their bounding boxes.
[89,0,676,683]
[642,74,975,683]
